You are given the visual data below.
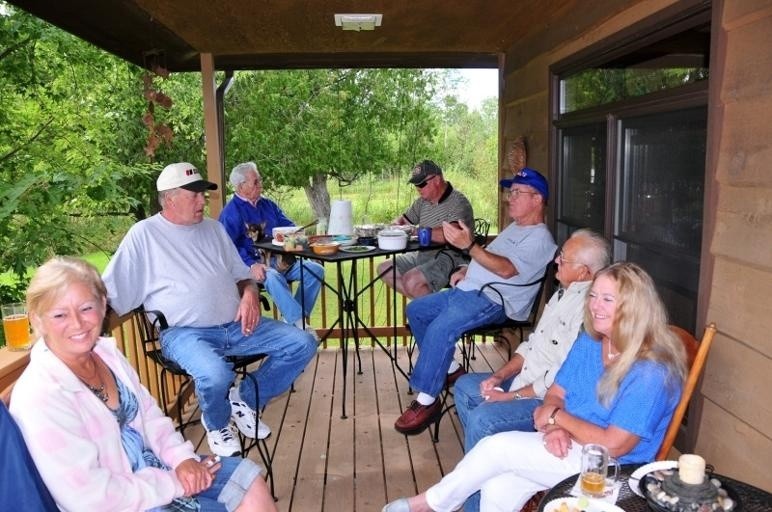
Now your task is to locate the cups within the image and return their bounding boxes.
[418,226,433,247]
[2,300,34,353]
[315,217,327,235]
[580,442,622,497]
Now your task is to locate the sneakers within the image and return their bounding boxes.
[201,411,243,458]
[443,363,467,385]
[394,395,442,433]
[293,323,322,342]
[228,386,272,439]
[381,497,411,511]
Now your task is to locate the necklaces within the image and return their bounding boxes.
[76,352,109,402]
[605,336,622,363]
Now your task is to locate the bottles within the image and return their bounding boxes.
[327,200,355,235]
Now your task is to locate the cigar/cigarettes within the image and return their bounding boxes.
[246,327,250,332]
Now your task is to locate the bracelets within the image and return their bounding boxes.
[514,391,521,399]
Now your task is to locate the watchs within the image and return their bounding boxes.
[462,240,475,255]
[548,406,560,425]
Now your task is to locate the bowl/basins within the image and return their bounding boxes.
[271,226,414,256]
[638,467,744,511]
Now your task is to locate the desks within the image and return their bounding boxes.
[537,462,771,511]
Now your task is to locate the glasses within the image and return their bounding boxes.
[415,175,436,189]
[504,188,543,202]
[557,251,584,267]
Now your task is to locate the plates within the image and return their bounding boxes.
[408,236,418,242]
[543,495,626,511]
[627,458,678,500]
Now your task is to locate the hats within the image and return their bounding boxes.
[499,168,549,199]
[156,162,218,192]
[408,160,442,184]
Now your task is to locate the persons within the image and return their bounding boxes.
[394,166,560,436]
[9,254,278,512]
[378,259,689,512]
[99,161,318,460]
[216,161,325,345]
[452,226,612,512]
[377,158,475,301]
